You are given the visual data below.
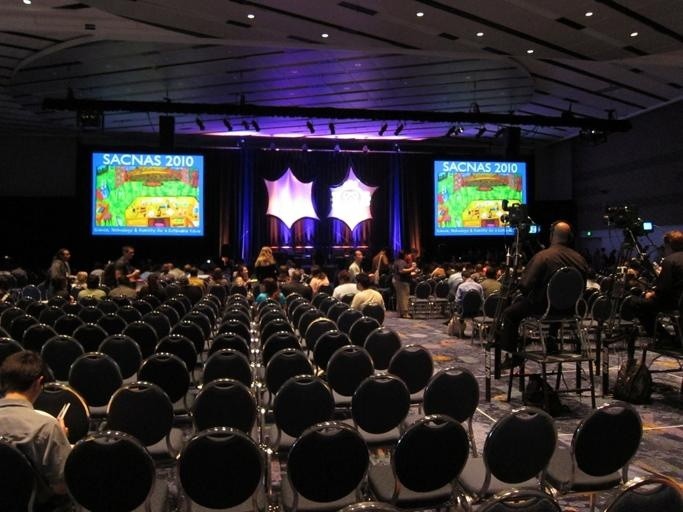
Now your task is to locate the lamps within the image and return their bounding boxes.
[241,122,488,141]
[222,118,233,132]
[195,116,206,131]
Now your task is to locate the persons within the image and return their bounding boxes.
[632,231,683,350]
[499,220,589,370]
[371,248,424,318]
[427,260,504,325]
[583,247,664,293]
[332,268,386,317]
[349,250,363,278]
[0,350,72,494]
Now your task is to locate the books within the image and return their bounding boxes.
[31,403,71,422]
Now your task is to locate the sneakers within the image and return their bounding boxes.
[647,337,677,350]
[500,352,528,370]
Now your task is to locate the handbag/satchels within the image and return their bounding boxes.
[614,358,653,405]
[524,374,571,417]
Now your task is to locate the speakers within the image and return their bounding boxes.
[504,126,520,158]
[159,115,175,145]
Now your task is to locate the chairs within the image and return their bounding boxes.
[0,268,683,512]
